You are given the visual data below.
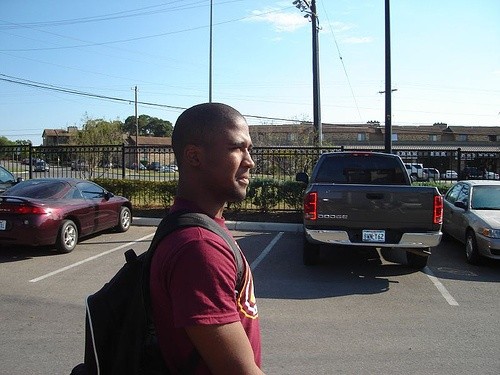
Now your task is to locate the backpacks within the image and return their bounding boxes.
[85,211,244,375]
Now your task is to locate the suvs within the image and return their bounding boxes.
[423,168,441,180]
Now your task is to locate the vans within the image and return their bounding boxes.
[405,162,423,182]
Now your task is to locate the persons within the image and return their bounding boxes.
[147,102,263,375]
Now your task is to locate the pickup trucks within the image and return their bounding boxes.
[294,151,443,270]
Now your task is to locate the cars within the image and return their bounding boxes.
[442,180,500,265]
[130,161,179,172]
[463,167,500,180]
[97,160,113,167]
[22,158,50,172]
[0,166,133,255]
[71,159,91,171]
[441,169,458,179]
